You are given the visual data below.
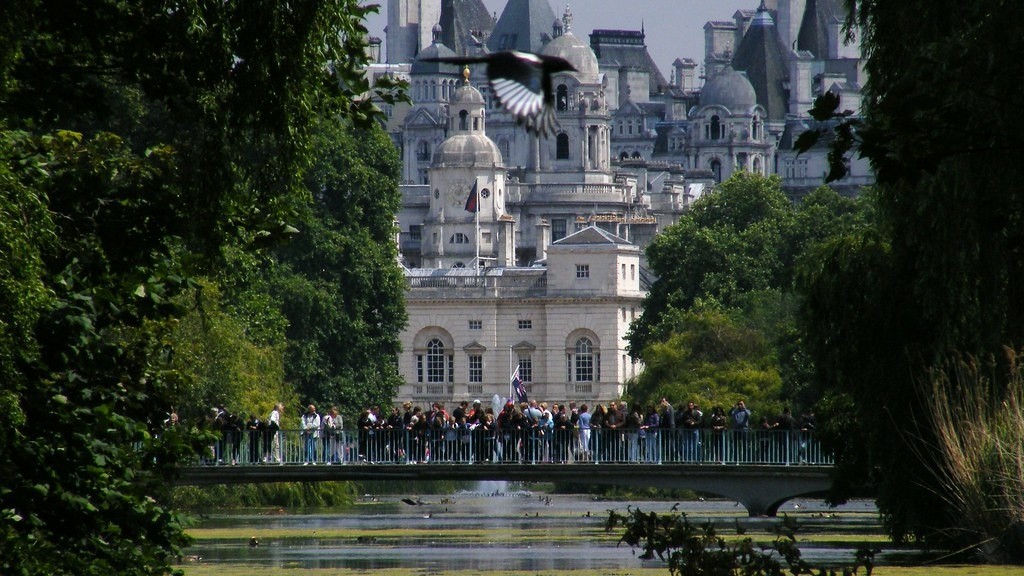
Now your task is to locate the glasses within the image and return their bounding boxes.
[610,405,614,406]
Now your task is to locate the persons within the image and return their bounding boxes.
[319,406,344,466]
[205,403,284,466]
[711,406,727,461]
[588,398,703,464]
[357,400,591,466]
[728,400,751,461]
[300,404,320,466]
[170,412,179,425]
[756,407,816,465]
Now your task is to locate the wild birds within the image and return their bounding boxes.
[416,48,584,139]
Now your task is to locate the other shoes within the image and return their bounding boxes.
[232,459,235,466]
[313,463,317,466]
[303,462,308,465]
[262,458,266,465]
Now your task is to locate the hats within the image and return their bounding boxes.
[505,402,513,408]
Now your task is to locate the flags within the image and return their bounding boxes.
[511,370,527,402]
[465,179,480,214]
